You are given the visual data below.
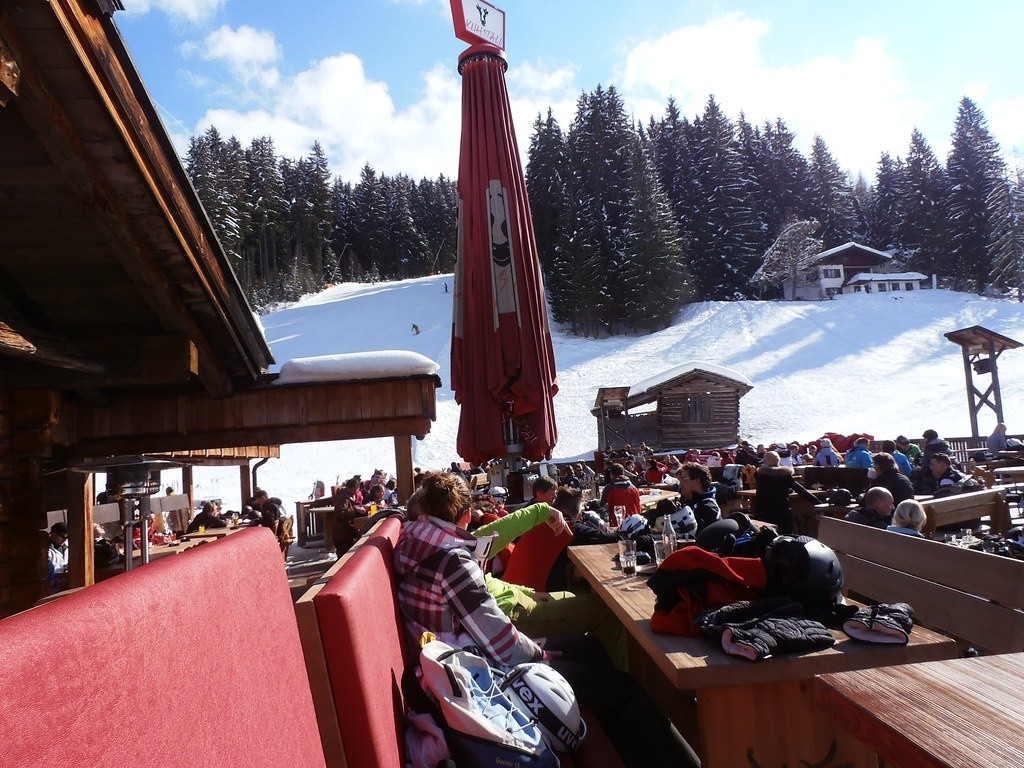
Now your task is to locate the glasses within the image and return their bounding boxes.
[619,532,628,541]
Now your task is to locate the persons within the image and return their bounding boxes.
[333,468,397,556]
[92,523,119,568]
[444,283,448,292]
[706,429,844,533]
[41,521,68,585]
[444,473,620,592]
[166,487,177,496]
[412,324,420,335]
[447,461,493,490]
[133,511,161,550]
[96,484,109,505]
[559,442,682,527]
[186,501,229,534]
[414,468,430,490]
[845,430,981,532]
[407,488,629,673]
[987,423,1007,453]
[621,462,721,551]
[394,469,625,768]
[244,488,286,535]
[886,499,927,538]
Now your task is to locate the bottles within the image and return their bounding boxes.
[662,514,677,557]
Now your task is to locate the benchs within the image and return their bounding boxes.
[2,458,1024,768]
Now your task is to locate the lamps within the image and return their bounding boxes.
[973,357,999,375]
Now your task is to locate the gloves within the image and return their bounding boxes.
[720,613,836,660]
[841,602,914,646]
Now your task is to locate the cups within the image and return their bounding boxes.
[233,513,238,524]
[945,529,977,544]
[654,541,665,568]
[867,468,875,478]
[614,505,626,528]
[619,540,636,578]
[198,525,205,533]
[152,533,177,545]
[370,504,377,516]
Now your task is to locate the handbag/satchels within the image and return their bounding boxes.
[646,546,765,637]
[418,631,561,768]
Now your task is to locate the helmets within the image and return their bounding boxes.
[656,504,697,539]
[830,488,851,506]
[488,486,508,504]
[497,662,587,751]
[618,512,649,539]
[695,510,778,558]
[764,534,843,617]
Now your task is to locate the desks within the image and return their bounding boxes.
[567,541,959,768]
[309,506,336,553]
[950,447,990,457]
[93,536,218,584]
[578,485,681,514]
[736,489,827,535]
[654,481,719,491]
[992,465,1024,484]
[814,494,935,521]
[181,525,246,540]
[808,652,1024,768]
